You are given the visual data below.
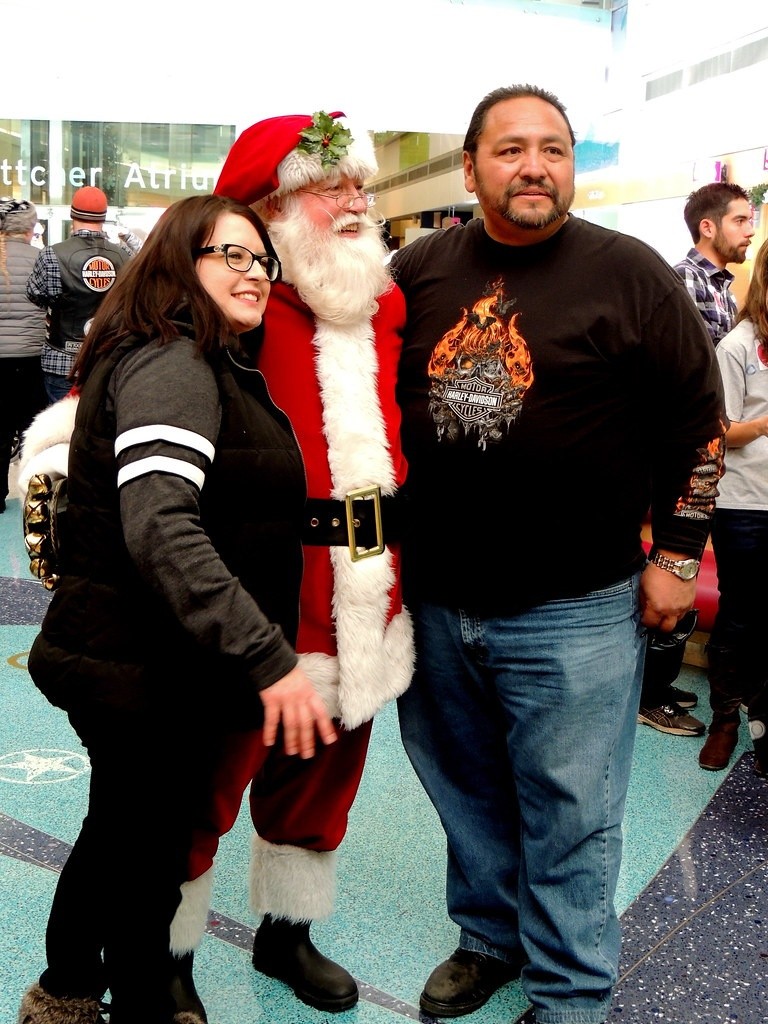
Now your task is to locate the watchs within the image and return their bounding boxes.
[649,549,700,581]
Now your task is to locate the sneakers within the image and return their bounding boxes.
[637,700,705,736]
[670,686,698,707]
[421,947,527,1015]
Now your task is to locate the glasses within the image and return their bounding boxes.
[296,190,380,208]
[194,244,281,283]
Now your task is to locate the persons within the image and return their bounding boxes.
[0,199,49,515]
[636,184,756,738]
[386,85,731,1024]
[16,199,339,1024]
[26,186,131,404]
[18,111,418,1013]
[698,239,768,776]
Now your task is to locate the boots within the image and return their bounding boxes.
[248,835,358,1010]
[698,693,742,769]
[168,856,207,1024]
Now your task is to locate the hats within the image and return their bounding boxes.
[213,111,378,209]
[70,186,108,222]
[0,199,38,231]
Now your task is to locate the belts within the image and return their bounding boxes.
[304,485,401,561]
